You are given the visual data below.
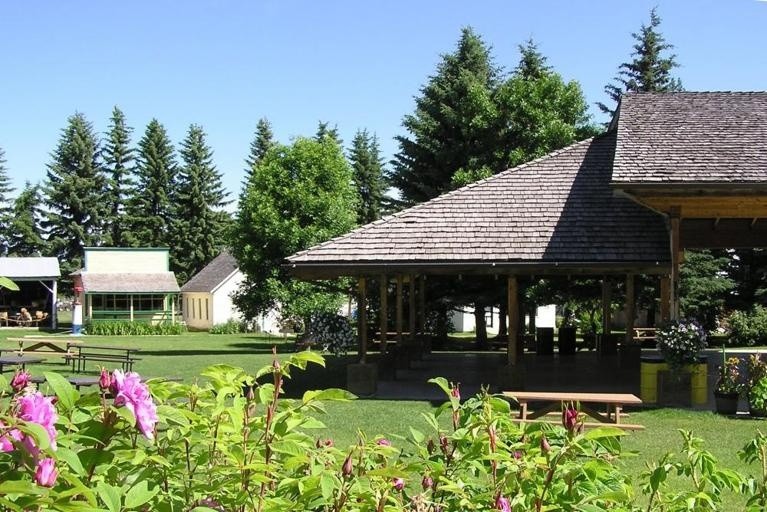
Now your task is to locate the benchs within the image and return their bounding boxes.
[509,408,631,423]
[505,415,644,445]
[0,338,141,390]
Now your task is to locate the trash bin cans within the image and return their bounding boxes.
[537,327,554,356]
[558,328,577,355]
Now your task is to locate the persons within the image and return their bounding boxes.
[15,305,32,326]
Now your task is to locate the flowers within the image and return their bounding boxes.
[712,353,766,410]
[655,318,714,376]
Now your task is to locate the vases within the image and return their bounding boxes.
[714,392,767,418]
[658,369,692,407]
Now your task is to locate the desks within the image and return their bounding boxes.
[502,390,644,447]
